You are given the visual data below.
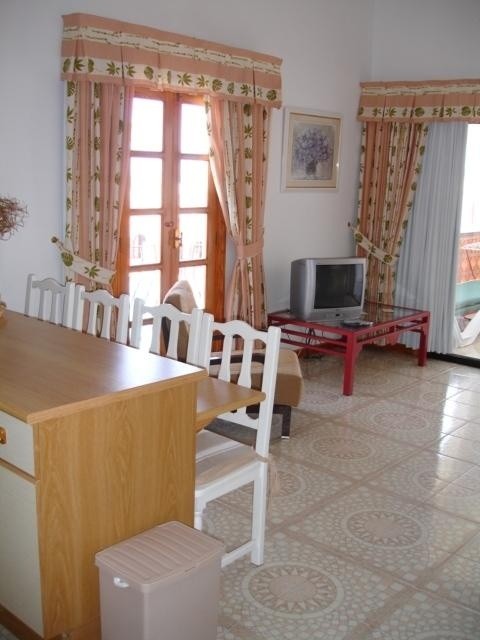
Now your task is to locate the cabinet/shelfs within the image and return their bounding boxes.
[0,307,267,640]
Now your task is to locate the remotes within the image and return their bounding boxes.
[341,321,371,327]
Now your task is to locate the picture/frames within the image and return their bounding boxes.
[280,106,344,194]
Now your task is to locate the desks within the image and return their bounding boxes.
[267,301,431,396]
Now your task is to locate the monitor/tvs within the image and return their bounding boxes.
[289,258,367,322]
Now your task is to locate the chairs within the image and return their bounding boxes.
[24,275,74,329]
[129,297,215,377]
[161,281,302,439]
[72,283,130,345]
[193,311,280,570]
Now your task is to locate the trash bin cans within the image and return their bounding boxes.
[95,521,225,640]
